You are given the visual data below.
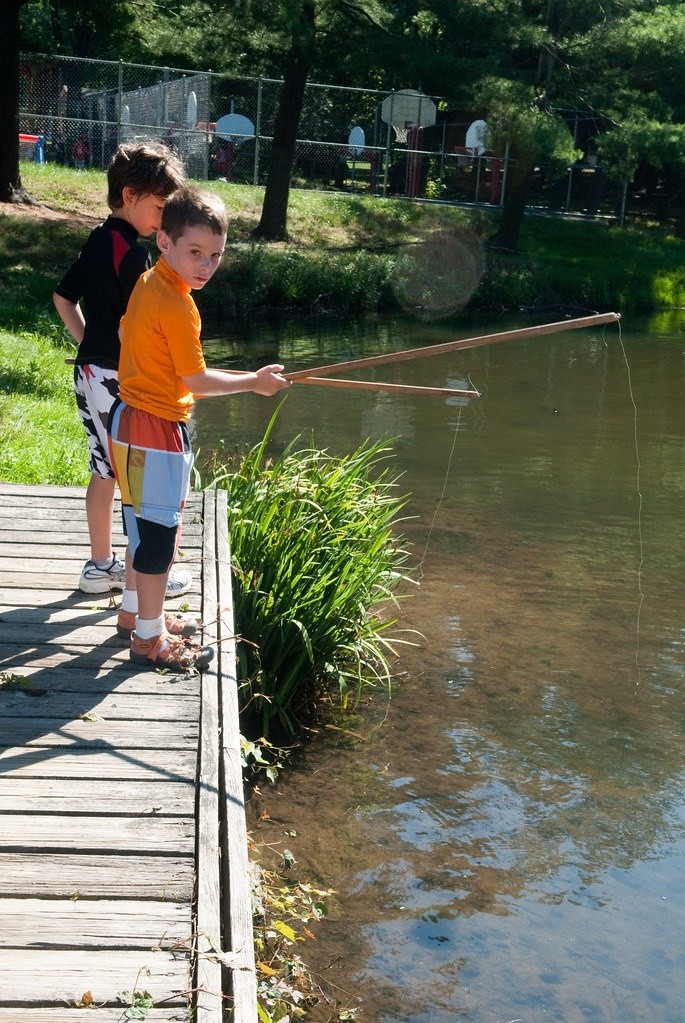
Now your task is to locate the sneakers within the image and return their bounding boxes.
[79,551,126,593]
[165,570,192,596]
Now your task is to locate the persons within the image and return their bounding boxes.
[53,134,66,165]
[71,137,90,168]
[53,145,193,599]
[107,185,292,670]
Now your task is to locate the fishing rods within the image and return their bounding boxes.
[282,309,620,383]
[209,365,482,401]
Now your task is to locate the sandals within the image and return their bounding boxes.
[129,630,214,667]
[117,608,198,640]
